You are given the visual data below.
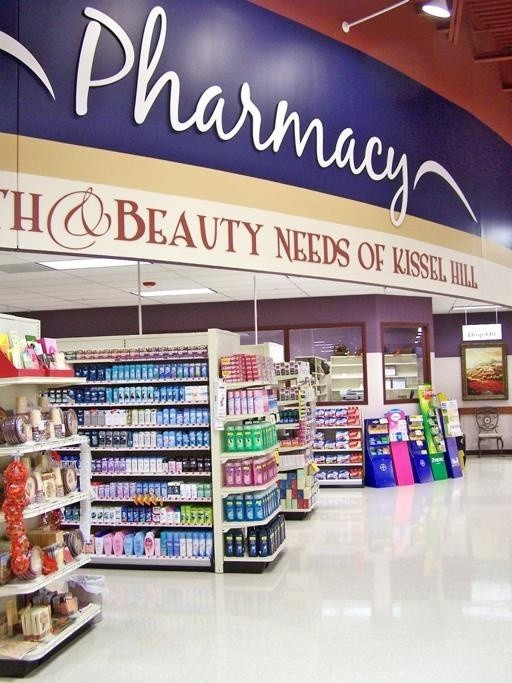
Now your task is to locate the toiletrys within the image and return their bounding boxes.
[41,349,286,558]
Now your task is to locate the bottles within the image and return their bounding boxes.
[43,367,87,403]
[61,500,81,522]
[60,454,77,470]
[20,452,65,500]
[17,395,63,441]
[267,408,303,425]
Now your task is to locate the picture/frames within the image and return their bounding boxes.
[460,339,510,401]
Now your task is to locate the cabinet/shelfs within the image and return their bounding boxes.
[362,415,461,490]
[384,352,421,399]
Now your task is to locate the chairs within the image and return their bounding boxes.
[475,405,505,458]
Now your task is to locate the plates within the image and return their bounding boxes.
[61,466,78,493]
[12,545,44,580]
[64,408,78,437]
[22,474,38,504]
[0,406,27,445]
[64,529,84,557]
